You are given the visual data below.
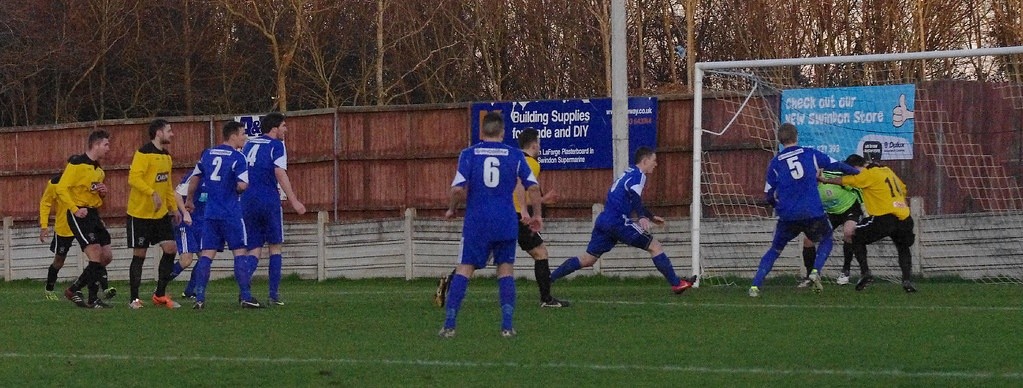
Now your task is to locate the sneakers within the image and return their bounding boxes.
[86,298,114,308]
[540,298,569,309]
[181,293,196,298]
[671,274,696,294]
[809,268,823,296]
[438,326,456,338]
[837,272,849,285]
[43,284,59,302]
[501,327,516,338]
[902,280,916,291]
[64,288,88,308]
[129,298,144,308]
[104,286,118,298]
[192,301,205,311]
[797,279,811,288]
[152,294,182,309]
[269,299,285,305]
[855,272,872,290]
[241,296,263,310]
[435,273,450,308]
[749,286,760,297]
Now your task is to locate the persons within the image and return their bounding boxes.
[127,118,181,310]
[438,113,542,339]
[749,122,860,298]
[56,129,113,309]
[436,129,570,309]
[798,154,919,293]
[40,155,117,301]
[239,113,306,307]
[550,146,697,295]
[153,121,266,309]
